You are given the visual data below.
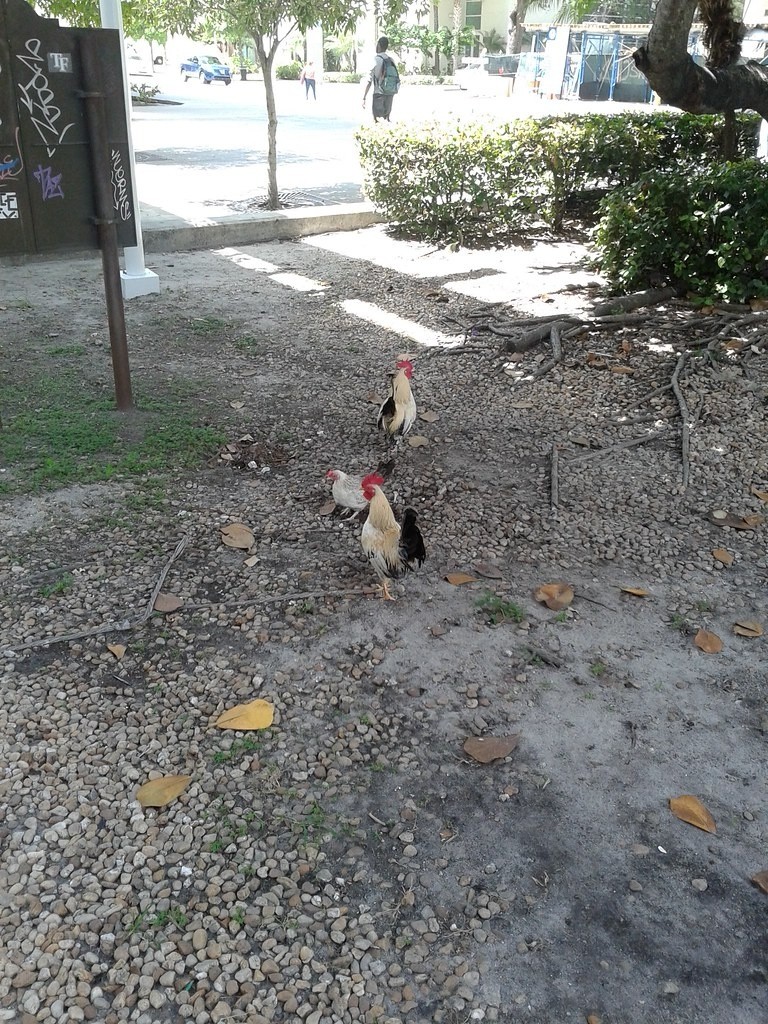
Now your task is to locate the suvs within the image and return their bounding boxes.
[460,53,545,92]
[180,55,232,85]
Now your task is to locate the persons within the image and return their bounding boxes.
[301,61,317,100]
[361,37,401,123]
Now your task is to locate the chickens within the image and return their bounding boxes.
[377,361,417,446]
[326,458,395,521]
[360,474,426,601]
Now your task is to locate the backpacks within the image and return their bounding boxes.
[373,55,400,95]
